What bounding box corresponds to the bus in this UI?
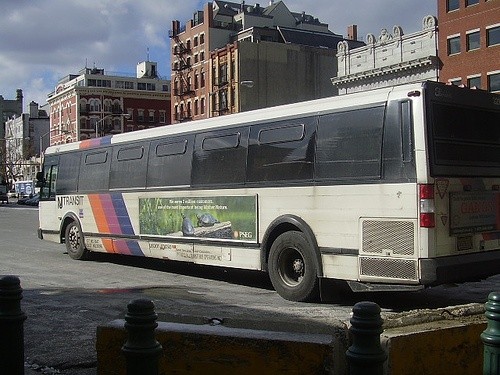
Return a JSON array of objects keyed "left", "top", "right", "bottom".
[{"left": 35, "top": 81, "right": 500, "bottom": 300}]
